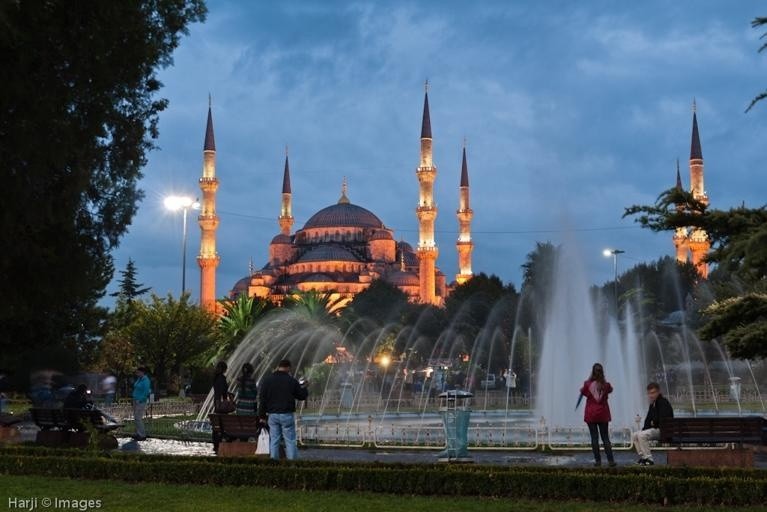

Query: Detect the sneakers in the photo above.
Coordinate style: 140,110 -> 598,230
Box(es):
636,458 -> 655,467
593,459 -> 618,468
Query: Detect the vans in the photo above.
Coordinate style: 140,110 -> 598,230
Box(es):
481,374 -> 497,389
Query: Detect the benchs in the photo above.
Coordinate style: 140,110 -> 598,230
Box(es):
207,412 -> 286,457
28,407 -> 126,447
656,417 -> 766,467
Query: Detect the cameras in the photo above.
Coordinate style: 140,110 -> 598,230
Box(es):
299,380 -> 306,385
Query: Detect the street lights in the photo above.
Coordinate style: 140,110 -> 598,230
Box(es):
163,195 -> 200,297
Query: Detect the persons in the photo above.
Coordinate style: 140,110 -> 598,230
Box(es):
502,368 -> 518,405
630,381 -> 674,468
131,367 -> 153,441
233,362 -> 258,414
258,360 -> 309,461
579,361 -> 619,467
211,360 -> 230,457
426,366 -> 466,406
100,370 -> 117,409
62,384 -> 88,426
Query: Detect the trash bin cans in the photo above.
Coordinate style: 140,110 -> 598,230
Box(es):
728,376 -> 742,402
340,383 -> 354,408
437,390 -> 474,464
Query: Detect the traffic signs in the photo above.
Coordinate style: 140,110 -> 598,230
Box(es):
602,248 -> 625,321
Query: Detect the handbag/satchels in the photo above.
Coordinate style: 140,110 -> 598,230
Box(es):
214,393 -> 237,414
